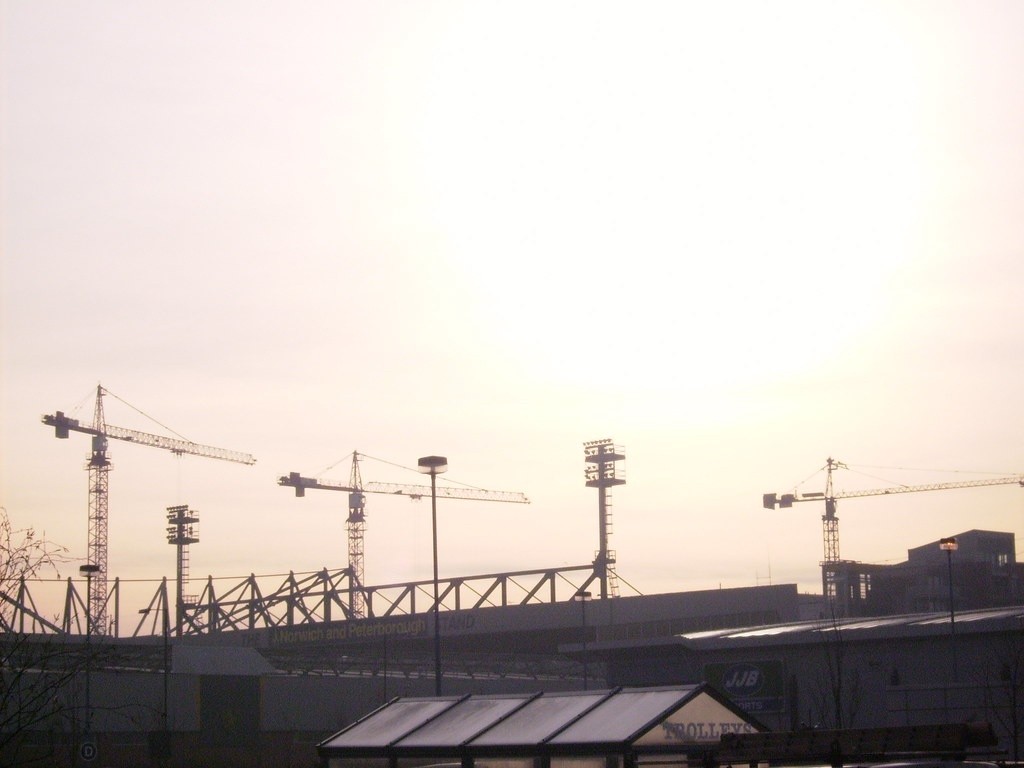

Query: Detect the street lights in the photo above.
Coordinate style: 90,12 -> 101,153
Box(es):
940,537 -> 960,680
75,563 -> 100,729
416,455 -> 449,694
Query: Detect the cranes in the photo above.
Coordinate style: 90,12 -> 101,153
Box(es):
44,382 -> 257,635
760,458 -> 1022,595
277,453 -> 533,622
